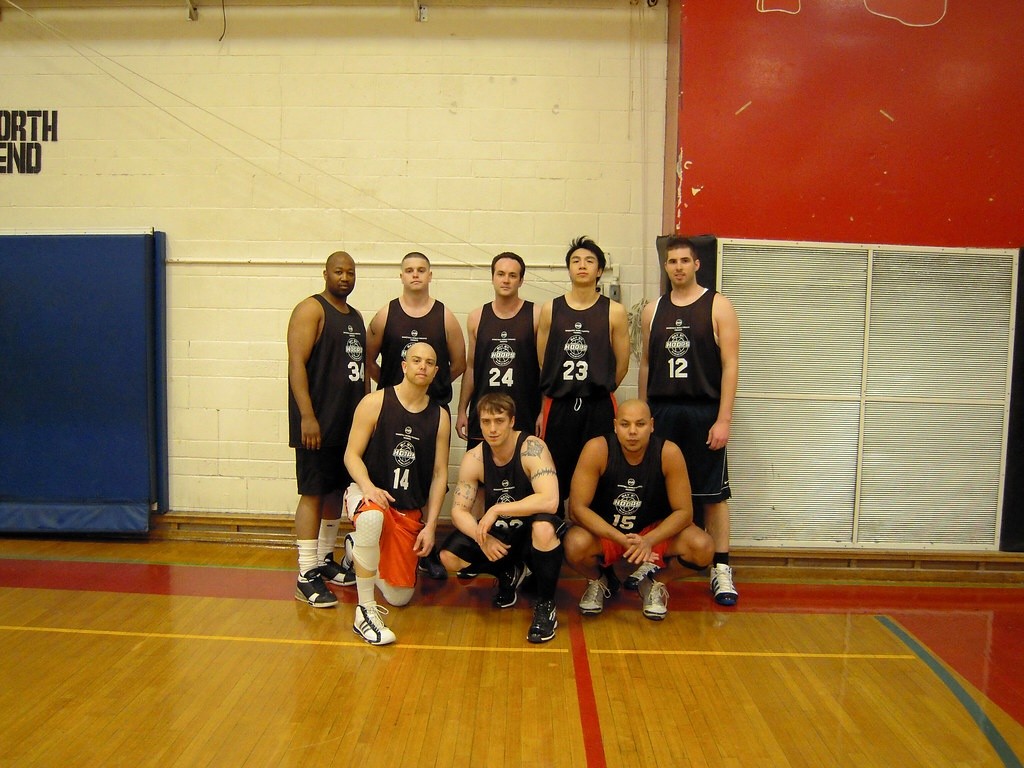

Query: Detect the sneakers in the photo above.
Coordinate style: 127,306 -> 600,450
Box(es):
527,596 -> 558,642
638,576 -> 670,621
319,551 -> 356,585
295,568 -> 338,607
493,561 -> 527,608
352,601 -> 396,645
419,547 -> 448,581
577,575 -> 610,616
623,561 -> 662,589
341,531 -> 358,572
601,567 -> 622,599
709,563 -> 738,605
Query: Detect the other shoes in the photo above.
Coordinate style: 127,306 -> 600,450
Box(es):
456,567 -> 478,578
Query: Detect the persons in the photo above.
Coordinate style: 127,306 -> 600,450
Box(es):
564,398 -> 715,620
341,341 -> 451,647
365,251 -> 467,577
455,253 -> 546,577
288,251 -> 372,610
439,392 -> 567,643
536,236 -> 739,606
